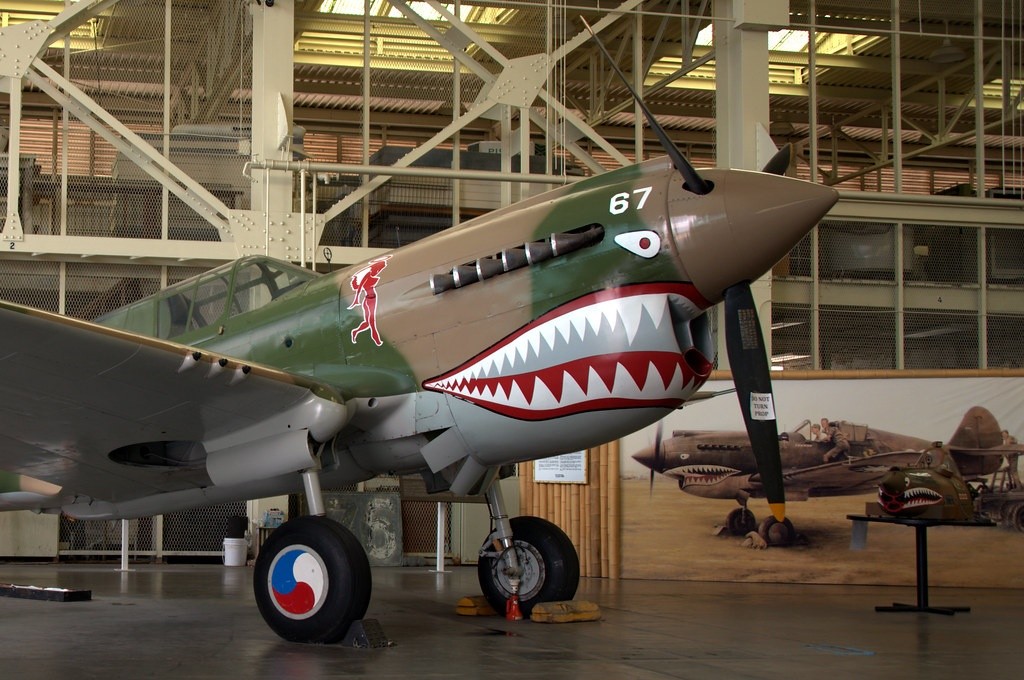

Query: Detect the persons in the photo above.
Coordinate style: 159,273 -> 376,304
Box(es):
812,418 -> 854,464
1002,430 -> 1023,492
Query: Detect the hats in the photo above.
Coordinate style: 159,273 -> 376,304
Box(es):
811,424 -> 820,429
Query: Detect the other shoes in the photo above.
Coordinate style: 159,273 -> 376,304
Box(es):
1011,487 -> 1024,492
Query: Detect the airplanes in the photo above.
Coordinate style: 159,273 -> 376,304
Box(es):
631,401 -> 1015,549
2,12 -> 843,647
